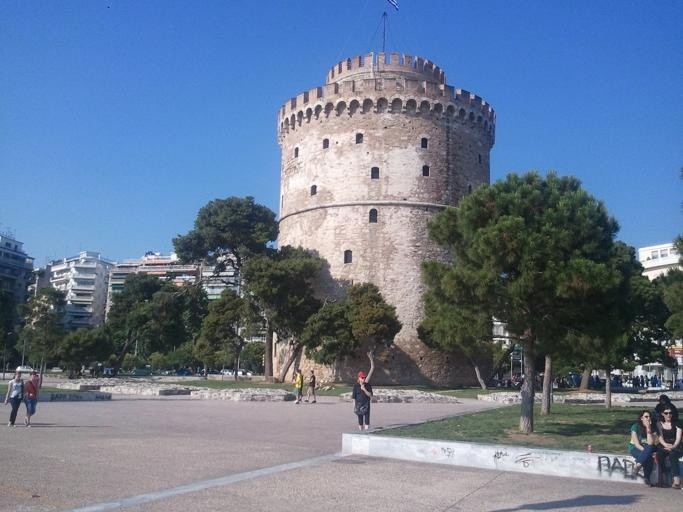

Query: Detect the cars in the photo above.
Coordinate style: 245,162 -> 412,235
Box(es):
16,366 -> 37,373
198,368 -> 253,376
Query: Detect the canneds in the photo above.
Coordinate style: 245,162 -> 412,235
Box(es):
587,444 -> 592,454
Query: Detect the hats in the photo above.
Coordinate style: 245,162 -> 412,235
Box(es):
359,372 -> 366,379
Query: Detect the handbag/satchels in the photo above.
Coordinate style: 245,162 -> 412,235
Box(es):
354,401 -> 369,415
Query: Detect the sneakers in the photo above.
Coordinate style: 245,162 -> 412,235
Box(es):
295,399 -> 316,404
645,480 -> 680,489
8,416 -> 31,427
633,466 -> 639,474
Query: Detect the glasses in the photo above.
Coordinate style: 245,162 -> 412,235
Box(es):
663,412 -> 672,415
645,416 -> 651,418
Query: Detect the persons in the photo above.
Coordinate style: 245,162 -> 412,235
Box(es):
653,394 -> 678,422
628,410 -> 654,488
632,374 -> 662,388
655,405 -> 683,488
351,370 -> 374,430
556,374 -> 601,388
22,371 -> 40,427
4,371 -> 24,427
291,370 -> 303,404
304,369 -> 317,404
